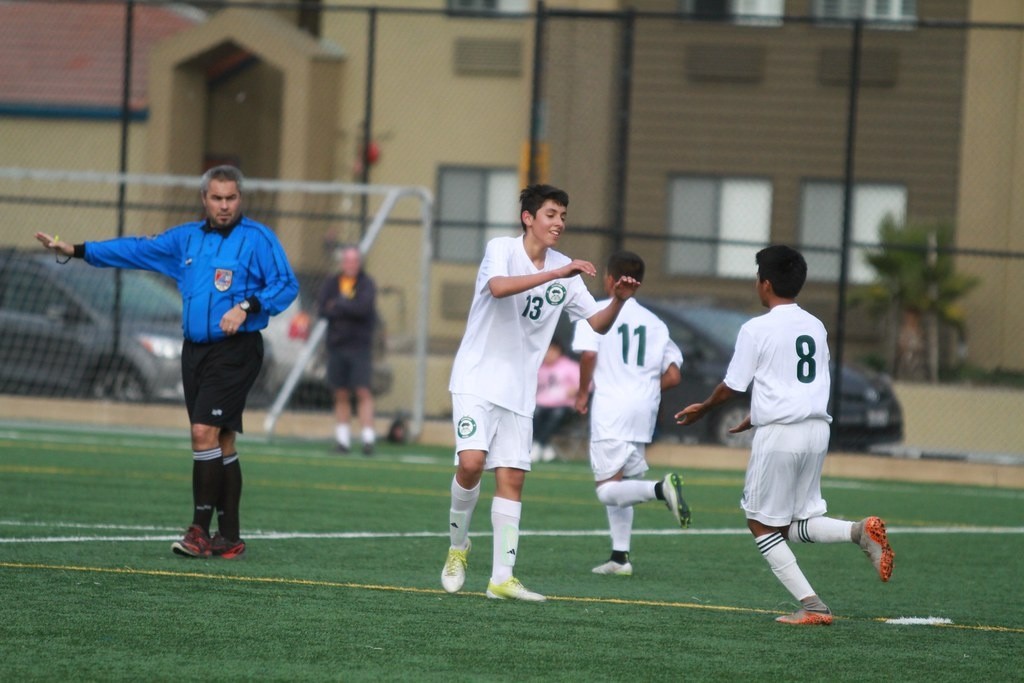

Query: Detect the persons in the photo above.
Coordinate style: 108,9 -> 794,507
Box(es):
531,338 -> 592,463
441,184 -> 641,602
34,165 -> 300,558
675,245 -> 894,624
573,250 -> 692,575
317,246 -> 377,456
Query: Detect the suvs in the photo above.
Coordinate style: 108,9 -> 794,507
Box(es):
259,270 -> 394,407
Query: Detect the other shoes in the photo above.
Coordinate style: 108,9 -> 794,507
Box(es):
530,441 -> 543,463
330,444 -> 351,454
362,443 -> 374,457
542,445 -> 556,463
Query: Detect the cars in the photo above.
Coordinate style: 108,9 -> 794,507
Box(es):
0,241 -> 278,409
551,295 -> 905,451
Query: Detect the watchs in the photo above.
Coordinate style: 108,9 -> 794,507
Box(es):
240,300 -> 250,311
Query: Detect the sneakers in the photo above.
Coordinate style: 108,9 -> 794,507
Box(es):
199,531 -> 246,559
857,516 -> 896,582
592,560 -> 632,576
662,473 -> 693,528
171,524 -> 212,558
440,537 -> 472,593
486,573 -> 546,603
775,607 -> 833,625
